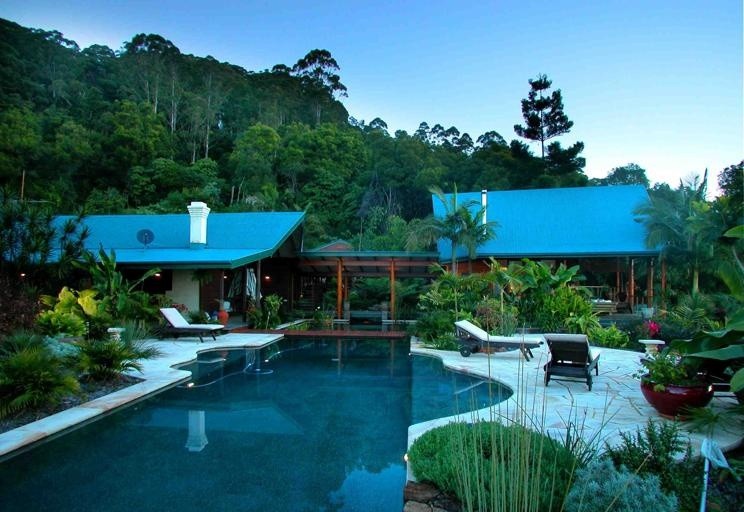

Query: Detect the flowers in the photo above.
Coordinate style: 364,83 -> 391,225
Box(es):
643,319 -> 661,339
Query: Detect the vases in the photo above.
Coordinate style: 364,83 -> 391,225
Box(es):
640,307 -> 654,318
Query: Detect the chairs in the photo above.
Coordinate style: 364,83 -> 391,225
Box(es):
452,317 -> 543,363
156,308 -> 224,344
541,333 -> 602,392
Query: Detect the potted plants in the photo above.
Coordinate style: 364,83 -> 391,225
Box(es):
637,353 -> 716,423
